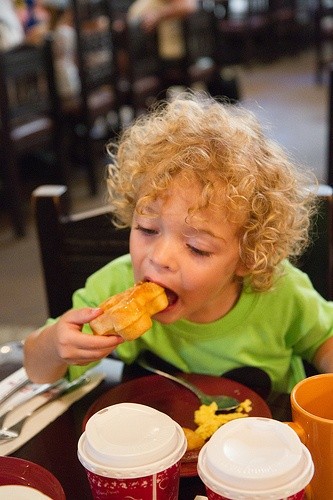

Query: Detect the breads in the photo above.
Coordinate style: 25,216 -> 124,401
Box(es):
89,282 -> 169,341
181,427 -> 206,450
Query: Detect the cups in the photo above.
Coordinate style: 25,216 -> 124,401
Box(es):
281,371 -> 333,500
196,416 -> 315,500
77,404 -> 188,500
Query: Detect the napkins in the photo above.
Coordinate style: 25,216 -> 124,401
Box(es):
0,366 -> 105,457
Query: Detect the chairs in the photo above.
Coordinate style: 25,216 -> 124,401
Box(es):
1,0 -> 333,243
31,185 -> 333,378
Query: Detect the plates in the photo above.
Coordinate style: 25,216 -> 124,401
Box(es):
82,369 -> 273,478
0,457 -> 65,500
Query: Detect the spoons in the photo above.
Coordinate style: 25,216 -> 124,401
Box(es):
135,357 -> 240,410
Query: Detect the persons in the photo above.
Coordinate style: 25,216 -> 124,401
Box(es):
0,0 -> 220,243
23,88 -> 333,421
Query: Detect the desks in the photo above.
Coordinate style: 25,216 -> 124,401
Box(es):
0,351 -> 292,500
